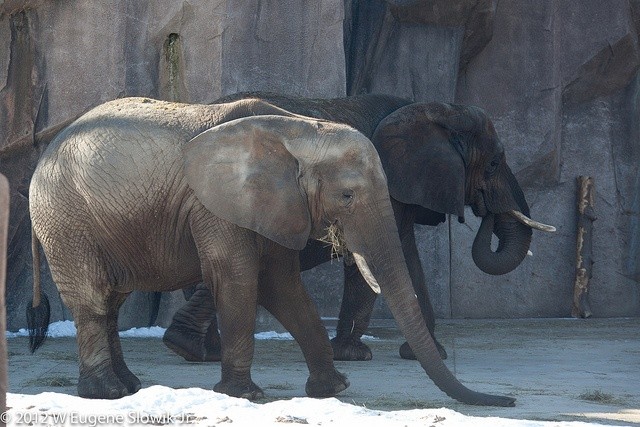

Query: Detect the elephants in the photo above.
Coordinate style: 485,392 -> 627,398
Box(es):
148,89 -> 561,362
25,93 -> 516,406
